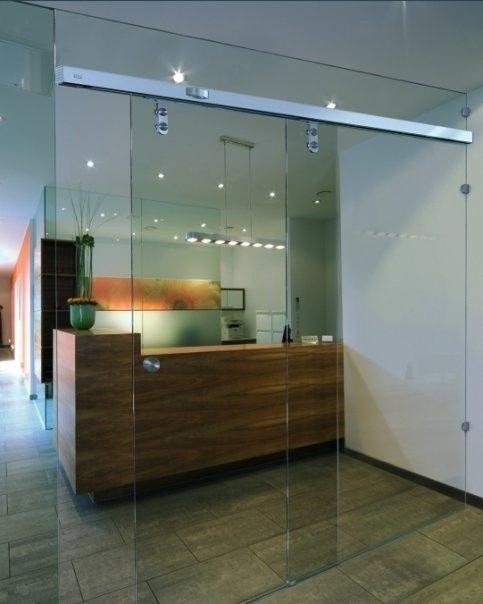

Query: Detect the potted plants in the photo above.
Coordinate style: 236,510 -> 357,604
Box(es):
67,234 -> 98,330
